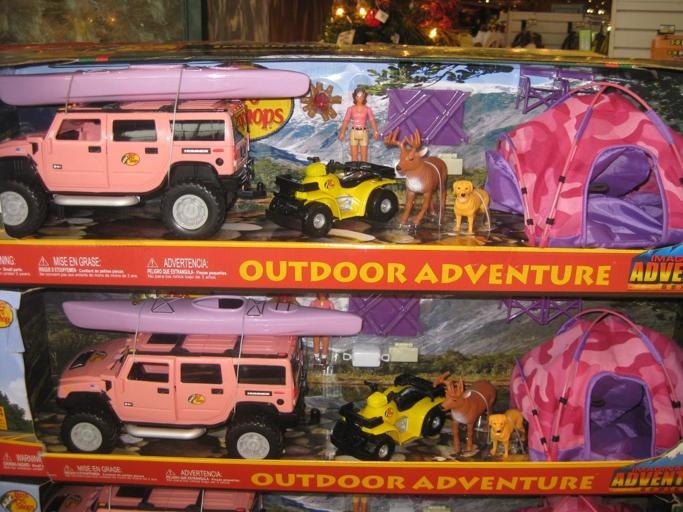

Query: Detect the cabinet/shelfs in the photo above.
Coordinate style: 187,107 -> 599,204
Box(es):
0,44 -> 683,511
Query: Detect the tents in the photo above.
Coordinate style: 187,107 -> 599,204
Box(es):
485,76 -> 683,247
509,306 -> 683,463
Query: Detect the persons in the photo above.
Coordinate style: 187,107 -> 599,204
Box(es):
309,293 -> 333,363
337,86 -> 378,162
